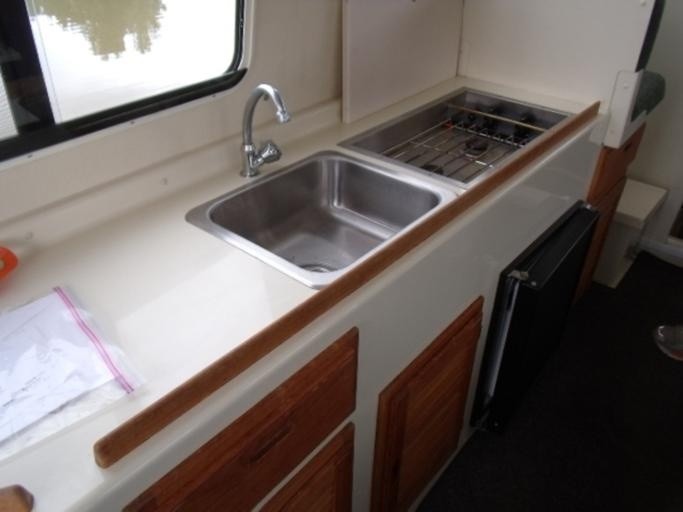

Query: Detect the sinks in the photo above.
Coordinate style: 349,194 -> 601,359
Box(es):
185,147 -> 460,288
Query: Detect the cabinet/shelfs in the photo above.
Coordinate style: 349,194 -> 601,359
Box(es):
118,325 -> 358,512
370,295 -> 484,512
570,121 -> 645,304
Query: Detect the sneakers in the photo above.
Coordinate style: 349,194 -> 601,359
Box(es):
651,324 -> 683,361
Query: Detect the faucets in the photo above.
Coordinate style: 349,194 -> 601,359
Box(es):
241,82 -> 290,145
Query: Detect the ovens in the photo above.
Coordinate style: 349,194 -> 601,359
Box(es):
474,203 -> 604,434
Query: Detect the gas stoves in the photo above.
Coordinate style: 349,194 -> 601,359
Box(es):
346,86 -> 566,186
